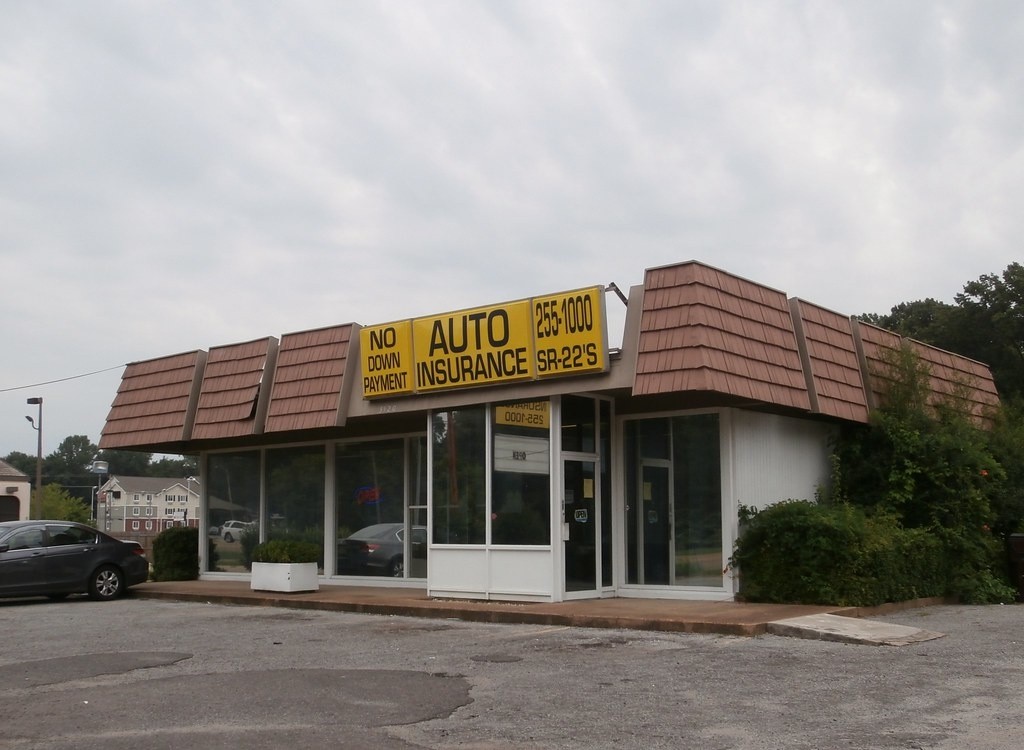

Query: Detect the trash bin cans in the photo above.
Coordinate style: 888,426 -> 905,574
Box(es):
1000,532 -> 1024,594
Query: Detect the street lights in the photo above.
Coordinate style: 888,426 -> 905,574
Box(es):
25,397 -> 43,519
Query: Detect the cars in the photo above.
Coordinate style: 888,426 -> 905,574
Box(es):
0,520 -> 149,601
340,522 -> 428,577
208,521 -> 252,543
